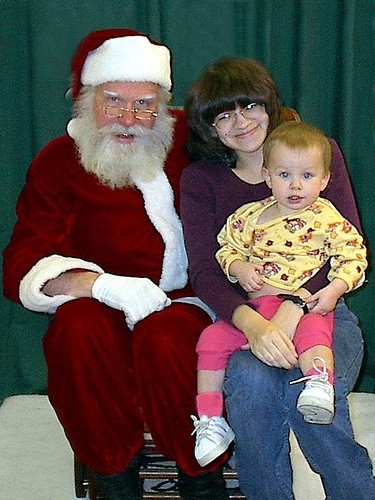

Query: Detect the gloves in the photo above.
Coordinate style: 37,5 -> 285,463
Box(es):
92,272 -> 171,330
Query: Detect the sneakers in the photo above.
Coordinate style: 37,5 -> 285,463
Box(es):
190,414 -> 235,467
290,357 -> 334,424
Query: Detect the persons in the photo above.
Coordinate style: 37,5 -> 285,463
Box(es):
190,121 -> 369,467
179,55 -> 375,499
1,29 -> 232,500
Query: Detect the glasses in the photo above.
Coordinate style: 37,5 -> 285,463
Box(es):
210,102 -> 265,131
95,103 -> 158,122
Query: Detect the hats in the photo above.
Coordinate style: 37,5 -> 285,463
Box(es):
66,27 -> 172,138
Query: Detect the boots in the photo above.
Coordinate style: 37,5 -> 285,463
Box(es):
176,465 -> 230,500
84,462 -> 145,500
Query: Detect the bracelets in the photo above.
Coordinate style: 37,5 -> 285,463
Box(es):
277,294 -> 308,314
